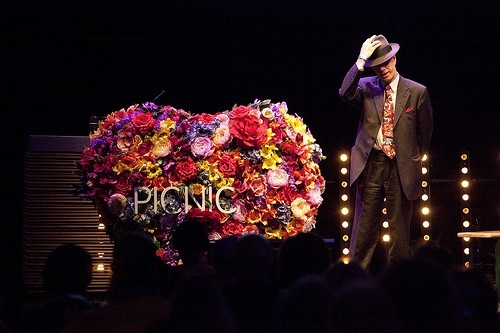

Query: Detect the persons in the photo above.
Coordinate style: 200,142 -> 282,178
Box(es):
0,220 -> 500,333
338,35 -> 432,271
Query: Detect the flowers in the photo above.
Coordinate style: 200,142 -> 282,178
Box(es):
74,100 -> 327,266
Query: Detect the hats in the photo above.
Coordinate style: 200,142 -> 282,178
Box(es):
365,34 -> 400,68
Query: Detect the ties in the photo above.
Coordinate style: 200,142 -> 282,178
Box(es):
382,84 -> 397,160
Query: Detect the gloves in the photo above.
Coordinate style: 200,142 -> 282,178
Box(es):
357,35 -> 382,61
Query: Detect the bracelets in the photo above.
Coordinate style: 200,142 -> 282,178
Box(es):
357,57 -> 367,62
